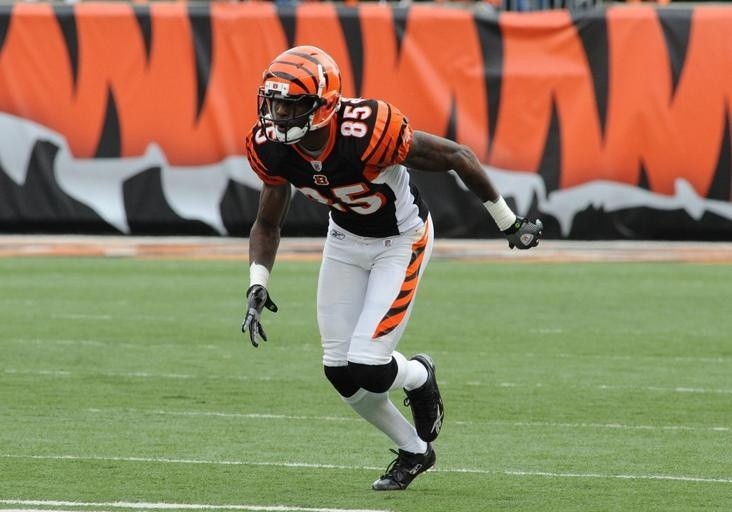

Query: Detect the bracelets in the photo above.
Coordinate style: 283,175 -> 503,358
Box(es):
484,196 -> 516,232
248,263 -> 270,289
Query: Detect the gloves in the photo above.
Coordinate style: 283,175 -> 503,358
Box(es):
500,216 -> 544,250
242,285 -> 277,348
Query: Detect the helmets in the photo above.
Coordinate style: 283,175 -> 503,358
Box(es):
255,43 -> 343,145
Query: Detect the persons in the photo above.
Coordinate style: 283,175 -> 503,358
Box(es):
241,45 -> 544,489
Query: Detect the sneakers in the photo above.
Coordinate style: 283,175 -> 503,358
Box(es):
402,353 -> 445,443
371,443 -> 437,490
